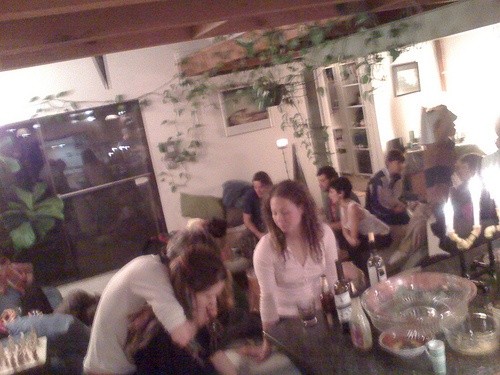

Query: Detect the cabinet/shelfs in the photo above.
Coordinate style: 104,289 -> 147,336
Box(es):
313,58 -> 389,193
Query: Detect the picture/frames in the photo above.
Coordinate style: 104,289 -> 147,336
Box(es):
219,83 -> 271,135
392,62 -> 421,98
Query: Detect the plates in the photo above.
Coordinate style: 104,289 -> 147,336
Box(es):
378,331 -> 434,356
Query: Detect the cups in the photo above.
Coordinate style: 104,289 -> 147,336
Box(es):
0,331 -> 39,371
295,297 -> 317,326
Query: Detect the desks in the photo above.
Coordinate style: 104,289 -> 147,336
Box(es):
263,238 -> 500,375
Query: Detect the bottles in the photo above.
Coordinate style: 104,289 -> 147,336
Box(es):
349,293 -> 372,355
366,232 -> 387,285
333,259 -> 353,331
319,274 -> 338,328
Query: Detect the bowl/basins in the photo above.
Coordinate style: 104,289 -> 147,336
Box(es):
438,306 -> 500,354
360,272 -> 477,338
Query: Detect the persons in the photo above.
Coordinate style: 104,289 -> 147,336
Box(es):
51,147 -> 140,231
0,151 -> 500,375
